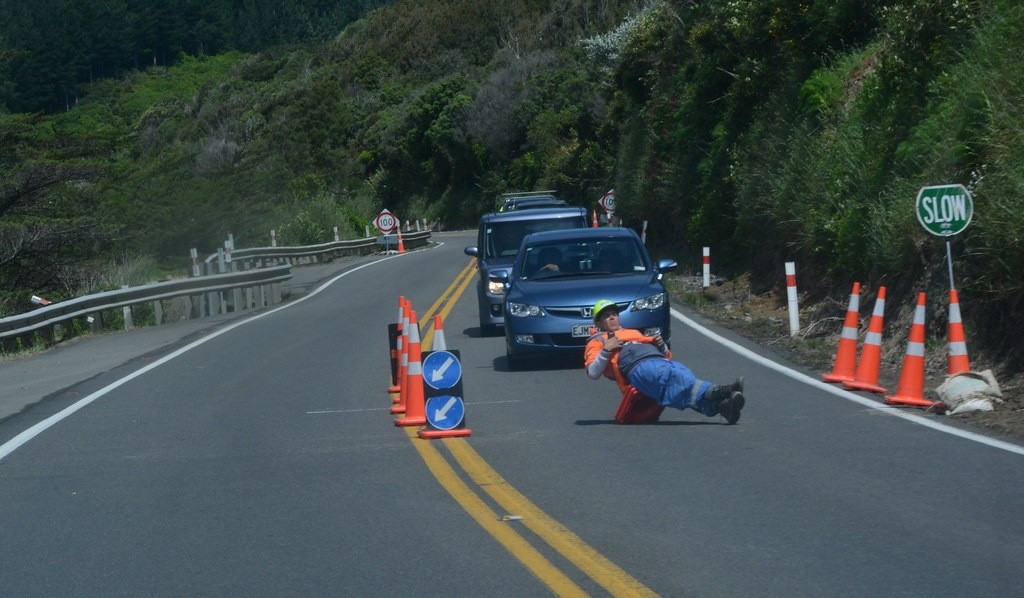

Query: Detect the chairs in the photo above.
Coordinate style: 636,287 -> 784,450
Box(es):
537,248 -> 563,270
597,248 -> 628,270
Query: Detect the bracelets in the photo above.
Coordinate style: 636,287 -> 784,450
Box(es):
603,348 -> 613,352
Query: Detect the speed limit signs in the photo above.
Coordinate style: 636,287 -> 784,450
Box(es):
372,208 -> 400,236
598,188 -> 617,217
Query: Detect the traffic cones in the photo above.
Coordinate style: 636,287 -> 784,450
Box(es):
592,210 -> 599,228
946,289 -> 970,375
397,229 -> 406,253
843,287 -> 888,394
416,314 -> 472,439
822,280 -> 861,384
885,291 -> 940,406
388,295 -> 425,428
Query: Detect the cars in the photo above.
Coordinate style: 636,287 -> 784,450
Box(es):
465,189 -> 588,335
488,225 -> 677,362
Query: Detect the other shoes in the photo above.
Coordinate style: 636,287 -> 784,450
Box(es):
711,376 -> 743,400
715,391 -> 745,424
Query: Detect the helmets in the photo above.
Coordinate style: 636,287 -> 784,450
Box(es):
593,300 -> 618,324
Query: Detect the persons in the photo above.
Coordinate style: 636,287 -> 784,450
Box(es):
584,299 -> 746,424
599,257 -> 614,271
536,247 -> 569,273
